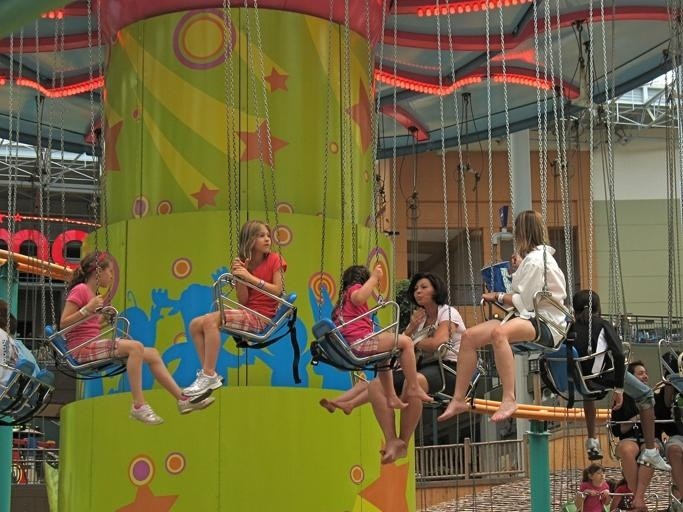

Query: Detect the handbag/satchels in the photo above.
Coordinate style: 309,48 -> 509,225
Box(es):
408,326 -> 447,364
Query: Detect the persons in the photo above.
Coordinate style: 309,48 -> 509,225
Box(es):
610,360 -> 659,512
332,265 -> 433,408
652,353 -> 679,460
367,273 -> 467,468
59,252 -> 215,424
564,290 -> 673,471
608,478 -> 635,512
579,464 -> 610,512
0,299 -> 54,424
654,351 -> 683,511
437,210 -> 569,421
319,380 -> 372,415
181,219 -> 288,397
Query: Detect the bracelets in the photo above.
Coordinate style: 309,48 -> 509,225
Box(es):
498,293 -> 507,304
255,278 -> 264,289
79,306 -> 92,318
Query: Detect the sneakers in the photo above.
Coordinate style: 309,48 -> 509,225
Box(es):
129,404 -> 163,425
586,438 -> 603,460
637,448 -> 672,471
178,369 -> 223,414
